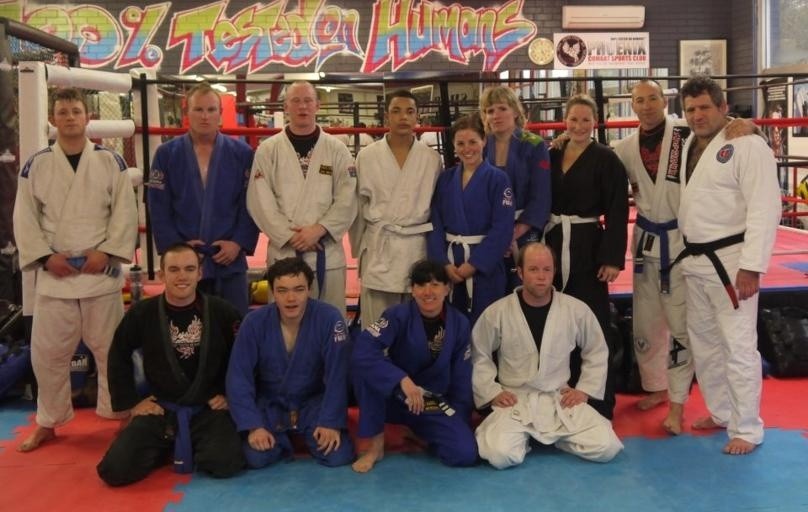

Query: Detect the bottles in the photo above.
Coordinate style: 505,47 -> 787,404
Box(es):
126,265 -> 143,304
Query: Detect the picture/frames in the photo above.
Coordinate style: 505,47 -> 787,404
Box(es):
679,38 -> 727,119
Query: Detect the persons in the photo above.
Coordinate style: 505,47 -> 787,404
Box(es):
427,115 -> 516,322
348,89 -> 444,330
346,259 -> 478,473
328,120 -> 374,155
678,79 -> 783,453
246,79 -> 356,321
469,241 -> 623,470
95,241 -> 247,486
13,88 -> 137,451
226,257 -> 357,468
546,94 -> 628,421
551,79 -> 769,434
480,85 -> 550,296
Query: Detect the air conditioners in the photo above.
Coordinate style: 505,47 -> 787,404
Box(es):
561,4 -> 645,30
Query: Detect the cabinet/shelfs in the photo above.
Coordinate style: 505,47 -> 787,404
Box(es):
1,17 -> 81,344
